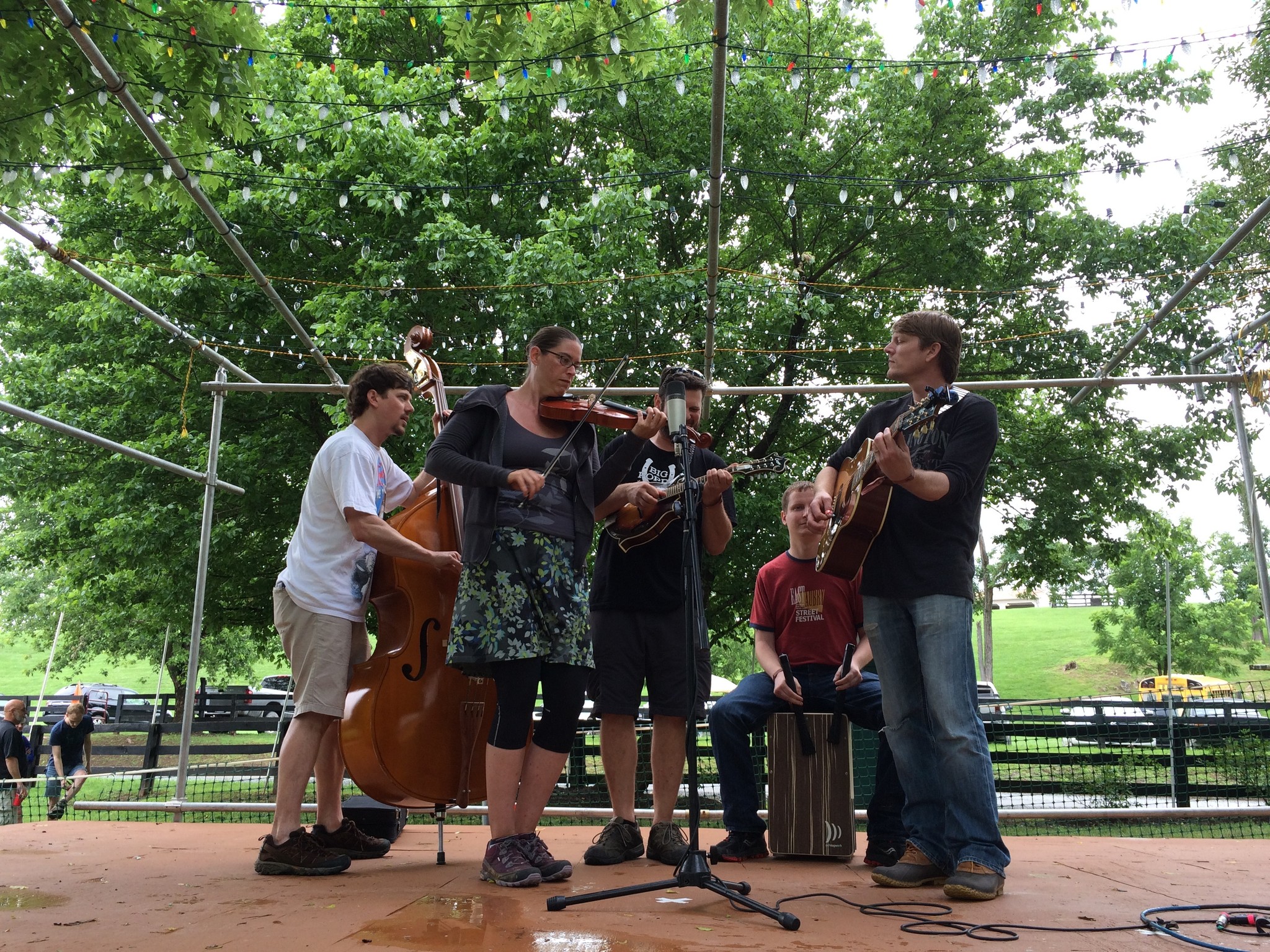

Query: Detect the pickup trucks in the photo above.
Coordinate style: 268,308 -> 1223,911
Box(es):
193,674 -> 296,735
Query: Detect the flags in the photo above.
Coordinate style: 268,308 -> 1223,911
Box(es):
72,680 -> 82,703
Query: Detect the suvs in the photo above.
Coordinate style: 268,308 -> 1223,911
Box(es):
42,682 -> 173,725
976,681 -> 1013,746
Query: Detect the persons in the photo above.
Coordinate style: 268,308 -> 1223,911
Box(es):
804,310 -> 1011,901
255,362 -> 462,874
583,365 -> 736,865
0,699 -> 34,826
424,327 -> 668,886
709,480 -> 910,866
43,703 -> 94,821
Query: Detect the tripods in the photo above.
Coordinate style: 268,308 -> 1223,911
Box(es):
545,433 -> 802,931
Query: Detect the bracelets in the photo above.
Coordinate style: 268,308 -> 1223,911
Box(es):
773,668 -> 783,682
893,468 -> 915,484
700,494 -> 723,509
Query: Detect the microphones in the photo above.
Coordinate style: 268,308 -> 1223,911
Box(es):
666,380 -> 687,458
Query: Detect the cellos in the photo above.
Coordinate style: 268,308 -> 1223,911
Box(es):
340,326 -> 538,864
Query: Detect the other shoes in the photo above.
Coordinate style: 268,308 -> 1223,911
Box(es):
46,803 -> 65,820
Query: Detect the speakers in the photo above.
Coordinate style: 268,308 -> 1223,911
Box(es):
340,795 -> 409,842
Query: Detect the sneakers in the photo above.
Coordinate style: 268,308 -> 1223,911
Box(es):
710,831 -> 769,860
480,834 -> 543,888
862,842 -> 908,868
583,817 -> 645,865
305,817 -> 390,859
253,827 -> 352,876
943,860 -> 1005,900
646,820 -> 690,866
871,843 -> 949,887
519,830 -> 573,881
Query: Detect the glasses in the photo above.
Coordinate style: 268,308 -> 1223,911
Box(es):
541,349 -> 583,372
14,707 -> 26,712
664,367 -> 706,380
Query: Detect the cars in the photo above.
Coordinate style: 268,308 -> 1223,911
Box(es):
1060,696 -> 1157,750
1181,698 -> 1267,719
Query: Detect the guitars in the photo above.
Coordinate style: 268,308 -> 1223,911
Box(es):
810,396 -> 942,585
597,450 -> 792,557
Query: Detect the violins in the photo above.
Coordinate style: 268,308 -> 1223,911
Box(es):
538,392 -> 713,451
